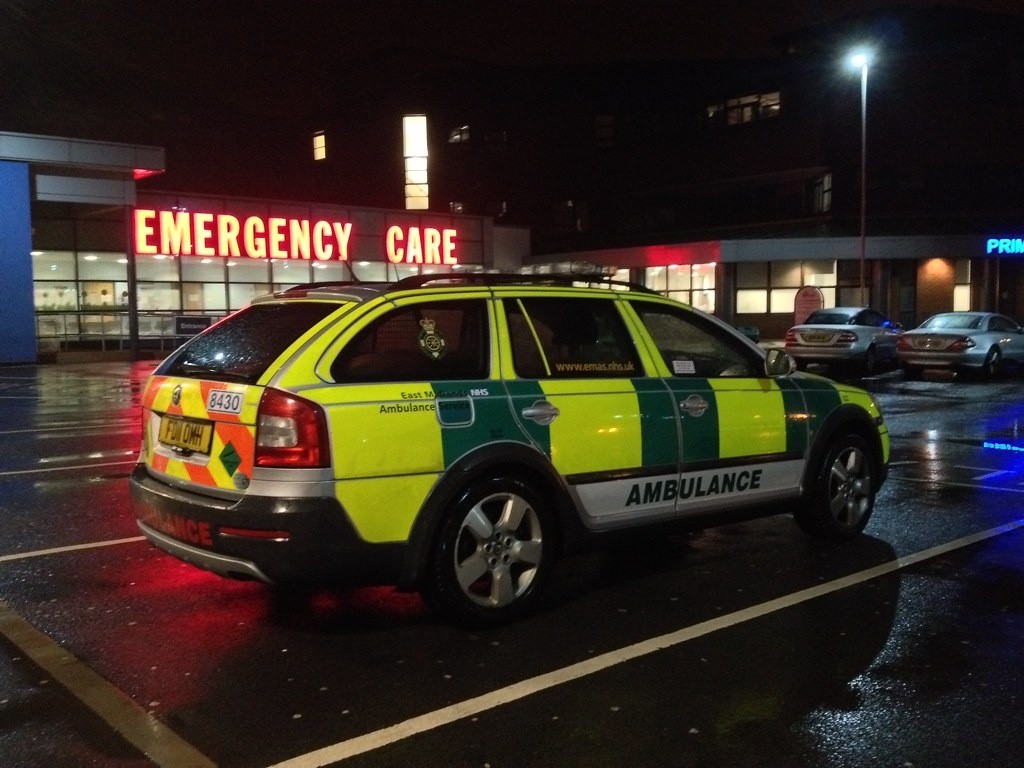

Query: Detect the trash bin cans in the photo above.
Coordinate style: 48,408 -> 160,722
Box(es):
738,326 -> 760,344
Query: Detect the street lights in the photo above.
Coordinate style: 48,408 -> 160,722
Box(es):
850,52 -> 870,309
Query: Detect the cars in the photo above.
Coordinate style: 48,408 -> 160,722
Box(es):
783,306 -> 908,371
895,310 -> 1024,377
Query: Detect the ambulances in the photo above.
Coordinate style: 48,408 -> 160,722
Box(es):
128,273 -> 894,623
